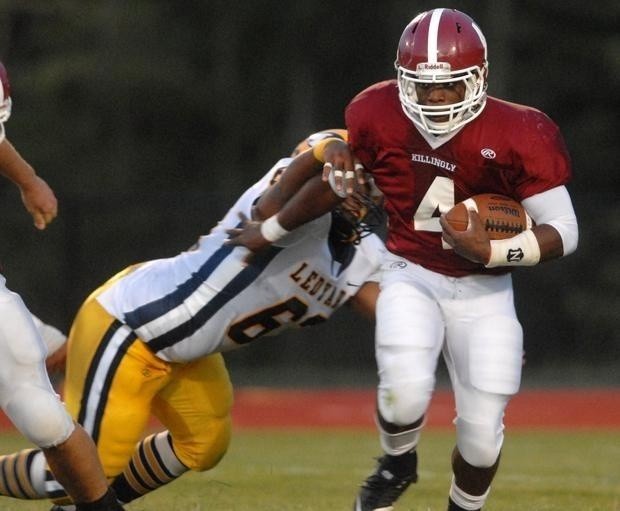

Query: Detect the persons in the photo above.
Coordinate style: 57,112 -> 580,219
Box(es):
0,61 -> 127,511
223,6 -> 584,511
0,128 -> 388,507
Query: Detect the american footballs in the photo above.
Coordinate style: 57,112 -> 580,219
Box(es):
445,193 -> 535,240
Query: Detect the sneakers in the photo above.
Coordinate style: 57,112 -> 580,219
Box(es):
352,446 -> 418,510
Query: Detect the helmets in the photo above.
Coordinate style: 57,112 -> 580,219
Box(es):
394,7 -> 489,135
275,127 -> 389,245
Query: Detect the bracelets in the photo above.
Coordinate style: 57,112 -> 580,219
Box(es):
260,212 -> 291,246
482,227 -> 540,268
